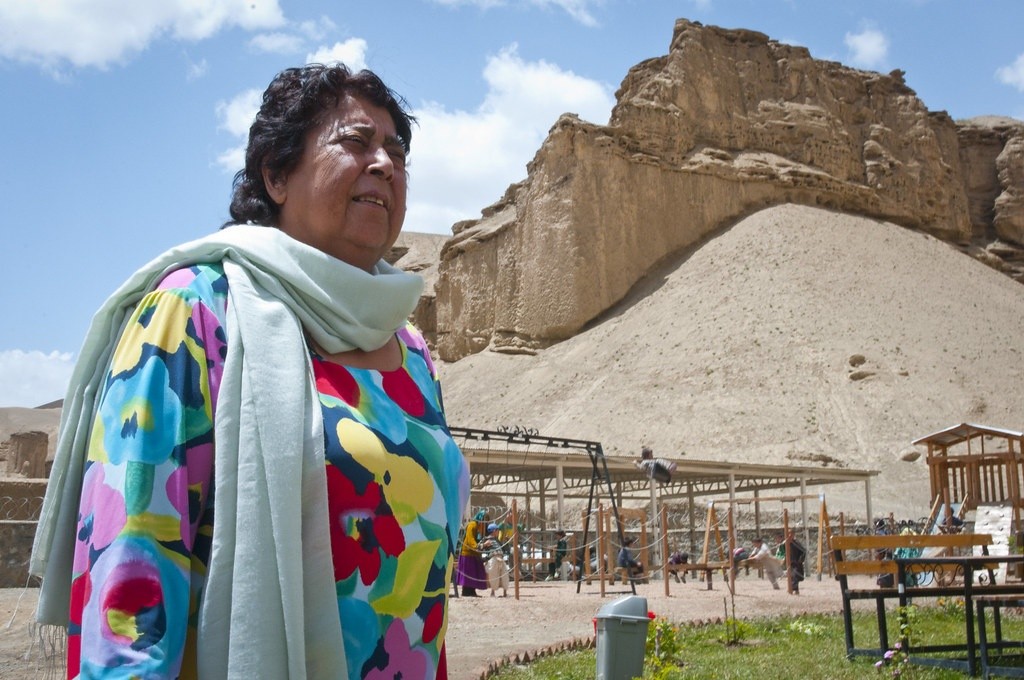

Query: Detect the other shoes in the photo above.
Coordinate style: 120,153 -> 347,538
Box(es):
681,577 -> 686,584
554,571 -> 560,579
545,575 -> 554,581
673,575 -> 680,584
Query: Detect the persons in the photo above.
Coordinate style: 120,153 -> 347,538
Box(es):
668,552 -> 689,584
634,449 -> 680,484
726,547 -> 749,575
875,519 -> 918,588
545,531 -> 567,581
741,535 -> 783,589
943,508 -> 962,527
457,509 -> 494,596
476,523 -> 509,596
777,529 -> 808,594
27,60 -> 472,679
616,536 -> 644,584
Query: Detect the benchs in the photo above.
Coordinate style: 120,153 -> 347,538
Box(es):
829,533 -> 1024,680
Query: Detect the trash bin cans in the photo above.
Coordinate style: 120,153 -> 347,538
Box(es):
591,595 -> 656,680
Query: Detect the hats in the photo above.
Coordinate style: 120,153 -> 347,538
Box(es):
487,523 -> 501,531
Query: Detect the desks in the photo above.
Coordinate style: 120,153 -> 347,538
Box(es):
893,553 -> 1024,678
